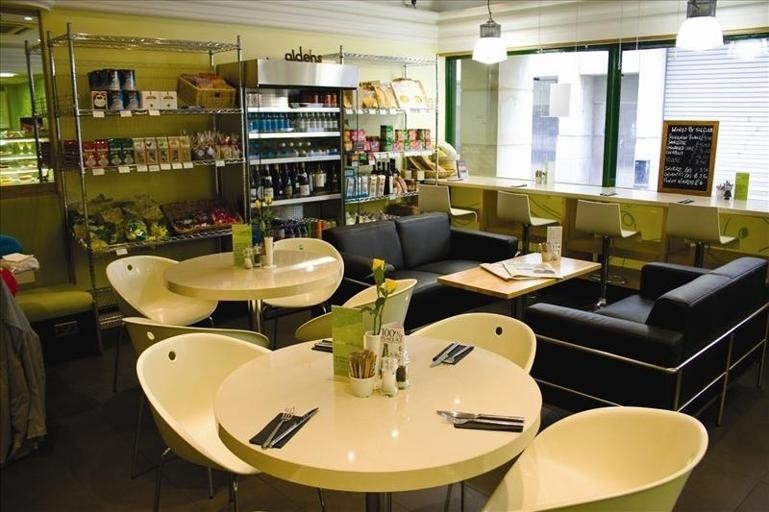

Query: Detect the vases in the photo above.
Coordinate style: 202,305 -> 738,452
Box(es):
262,235 -> 274,266
361,330 -> 381,392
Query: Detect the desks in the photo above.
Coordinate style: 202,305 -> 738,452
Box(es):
213,336 -> 545,512
438,252 -> 603,324
160,248 -> 342,331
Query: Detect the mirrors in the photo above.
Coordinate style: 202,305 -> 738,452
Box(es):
0,6 -> 58,203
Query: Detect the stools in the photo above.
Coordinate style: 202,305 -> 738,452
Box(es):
575,198 -> 639,312
132,329 -> 328,511
666,200 -> 741,269
494,189 -> 560,255
416,183 -> 479,232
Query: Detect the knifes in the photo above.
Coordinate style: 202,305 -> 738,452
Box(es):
269,406 -> 320,447
429,343 -> 460,369
436,409 -> 524,423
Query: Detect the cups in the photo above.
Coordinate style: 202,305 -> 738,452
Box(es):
348,371 -> 376,397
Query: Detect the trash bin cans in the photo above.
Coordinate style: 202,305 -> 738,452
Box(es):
634,160 -> 650,184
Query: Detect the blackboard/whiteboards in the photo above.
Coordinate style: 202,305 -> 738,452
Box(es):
657,121 -> 719,197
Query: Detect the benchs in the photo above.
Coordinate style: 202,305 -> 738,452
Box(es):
12,282 -> 95,324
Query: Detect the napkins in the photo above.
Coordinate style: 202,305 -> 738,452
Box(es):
250,412 -> 307,451
431,341 -> 475,367
312,338 -> 332,355
453,411 -> 527,435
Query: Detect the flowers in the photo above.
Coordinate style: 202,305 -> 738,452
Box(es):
252,197 -> 276,233
353,254 -> 398,333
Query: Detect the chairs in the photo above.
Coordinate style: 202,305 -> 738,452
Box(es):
405,306 -> 539,512
479,402 -> 712,512
249,236 -> 346,337
290,277 -> 420,339
120,315 -> 271,480
104,253 -> 217,393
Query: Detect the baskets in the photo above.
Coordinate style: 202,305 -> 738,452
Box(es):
410,168 -> 455,178
177,76 -> 237,108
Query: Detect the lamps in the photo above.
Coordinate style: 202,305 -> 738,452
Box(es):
473,1 -> 509,65
675,1 -> 725,52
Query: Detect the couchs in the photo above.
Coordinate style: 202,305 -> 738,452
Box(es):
318,211 -> 519,333
520,255 -> 768,428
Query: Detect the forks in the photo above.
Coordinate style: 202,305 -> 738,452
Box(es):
439,344 -> 473,364
441,411 -> 528,425
261,404 -> 296,449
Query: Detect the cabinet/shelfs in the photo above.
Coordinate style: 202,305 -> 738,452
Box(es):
47,21 -> 248,356
307,45 -> 439,227
0,130 -> 50,179
216,44 -> 358,320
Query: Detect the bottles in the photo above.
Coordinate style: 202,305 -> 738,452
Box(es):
243,244 -> 262,269
371,161 -> 393,194
382,346 -> 410,396
248,112 -> 339,239
